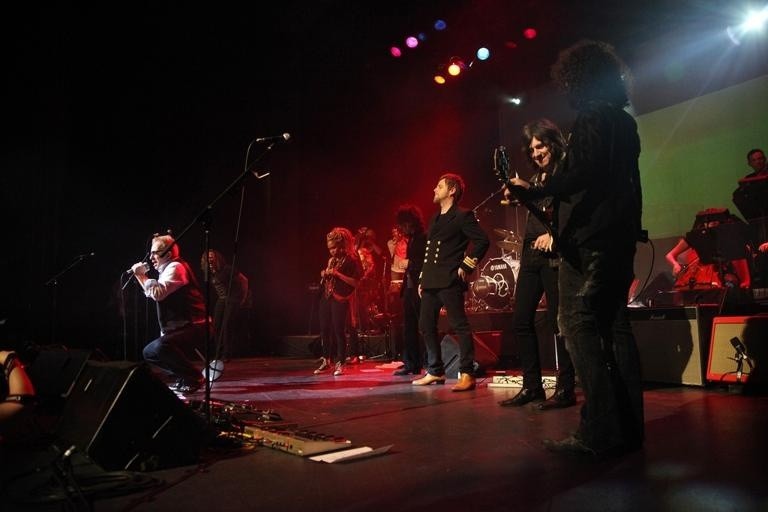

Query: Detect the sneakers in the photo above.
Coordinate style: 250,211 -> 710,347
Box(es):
167,376 -> 206,393
313,360 -> 334,375
333,361 -> 346,375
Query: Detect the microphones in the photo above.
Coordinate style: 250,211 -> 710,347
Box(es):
80,252 -> 95,258
126,262 -> 150,275
256,133 -> 291,144
730,336 -> 750,362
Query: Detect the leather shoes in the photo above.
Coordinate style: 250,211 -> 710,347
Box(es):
411,372 -> 446,386
498,388 -> 546,408
451,373 -> 476,391
538,392 -> 577,410
394,361 -> 424,376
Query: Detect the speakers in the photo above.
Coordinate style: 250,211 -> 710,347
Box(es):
626,304 -> 719,387
706,313 -> 768,385
51,368 -> 206,468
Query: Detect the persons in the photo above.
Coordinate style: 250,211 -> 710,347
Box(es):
132,235 -> 216,397
313,227 -> 365,376
346,225 -> 411,367
498,118 -> 576,411
542,39 -> 645,457
665,209 -> 740,290
0,350 -> 35,419
201,249 -> 249,363
396,210 -> 428,376
411,173 -> 490,392
733,149 -> 768,221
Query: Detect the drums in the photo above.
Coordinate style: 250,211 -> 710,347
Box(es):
481,256 -> 521,310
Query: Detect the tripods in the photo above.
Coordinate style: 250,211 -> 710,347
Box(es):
195,231 -> 240,448
345,289 -> 377,357
368,281 -> 400,360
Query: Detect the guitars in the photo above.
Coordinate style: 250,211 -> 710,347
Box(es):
494,146 -> 552,235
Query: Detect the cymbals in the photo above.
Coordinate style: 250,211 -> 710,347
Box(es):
494,228 -> 522,242
496,240 -> 522,253
